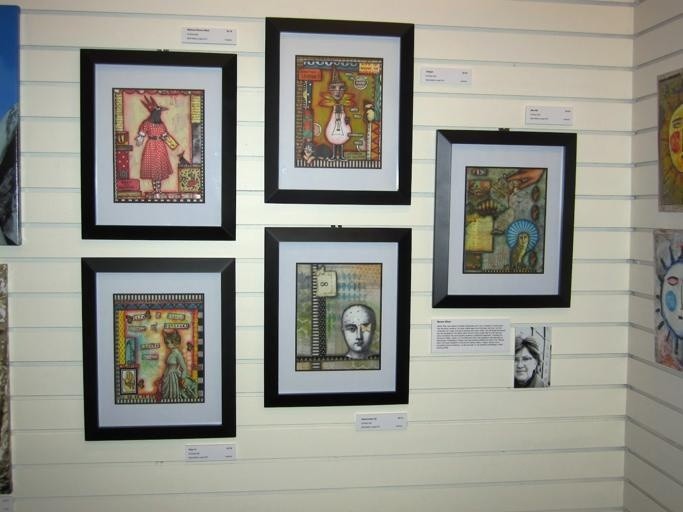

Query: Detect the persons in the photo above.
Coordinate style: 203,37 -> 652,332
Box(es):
514,336 -> 547,388
340,303 -> 378,360
156,328 -> 198,399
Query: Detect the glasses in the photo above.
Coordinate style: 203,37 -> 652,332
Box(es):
514,357 -> 533,362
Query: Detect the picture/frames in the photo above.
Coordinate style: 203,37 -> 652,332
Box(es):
264,16 -> 415,206
262,225 -> 413,409
0,265 -> 14,492
431,130 -> 579,308
80,48 -> 237,241
80,255 -> 237,443
0,4 -> 21,244
